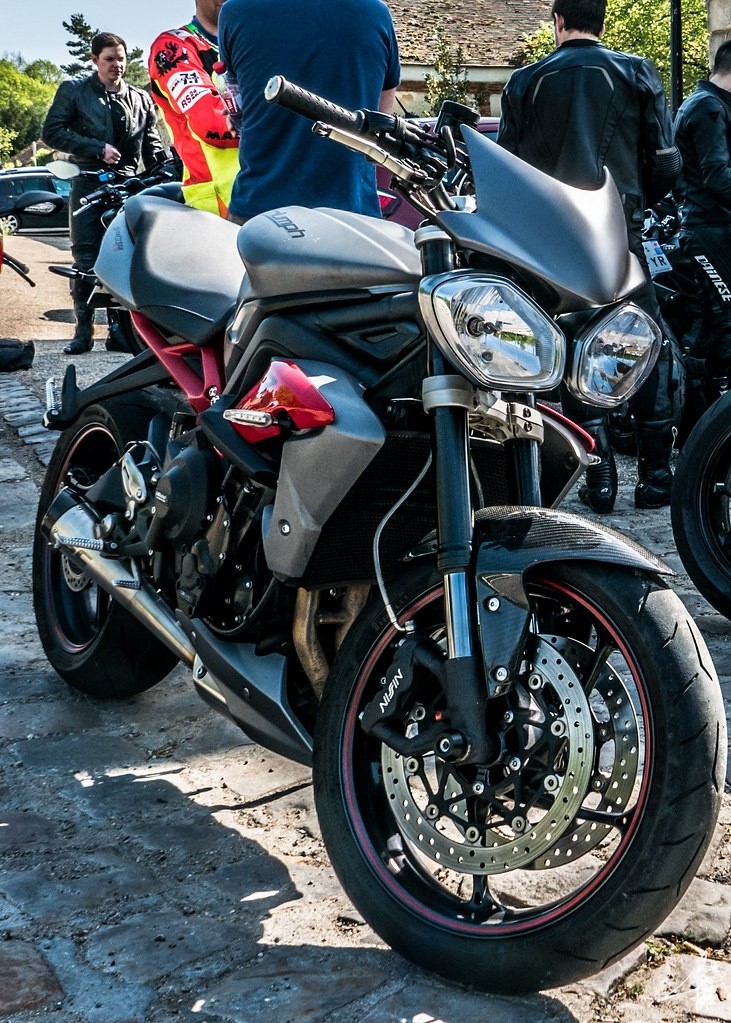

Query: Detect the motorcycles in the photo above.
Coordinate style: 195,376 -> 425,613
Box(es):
44,153 -> 183,356
31,76 -> 728,994
608,194 -> 731,623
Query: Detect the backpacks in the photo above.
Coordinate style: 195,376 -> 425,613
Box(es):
0,338 -> 35,372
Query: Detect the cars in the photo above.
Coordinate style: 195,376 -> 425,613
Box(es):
375,117 -> 500,231
0,167 -> 70,236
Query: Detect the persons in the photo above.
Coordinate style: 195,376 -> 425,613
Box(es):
670,38 -> 731,435
217,0 -> 401,226
146,0 -> 241,219
42,31 -> 167,355
495,0 -> 685,512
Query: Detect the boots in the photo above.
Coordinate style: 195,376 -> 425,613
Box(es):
633,419 -> 679,509
104,303 -> 144,353
63,303 -> 97,354
575,419 -> 619,513
